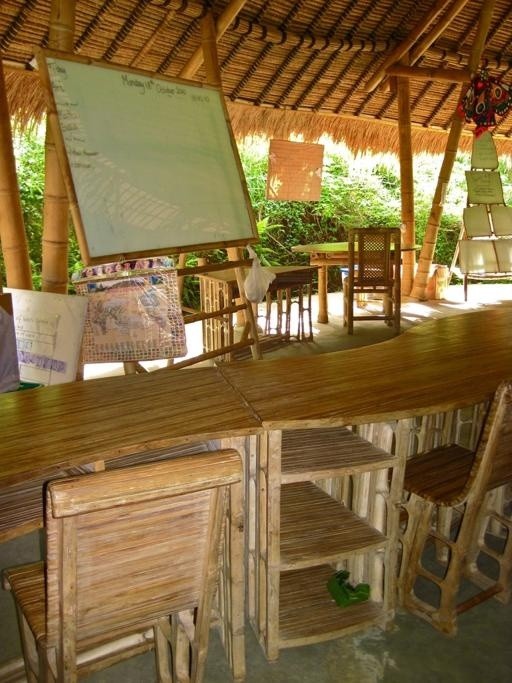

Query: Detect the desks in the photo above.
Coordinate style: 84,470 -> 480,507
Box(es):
206,241 -> 422,341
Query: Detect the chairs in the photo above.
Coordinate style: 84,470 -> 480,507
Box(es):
345,227 -> 404,338
